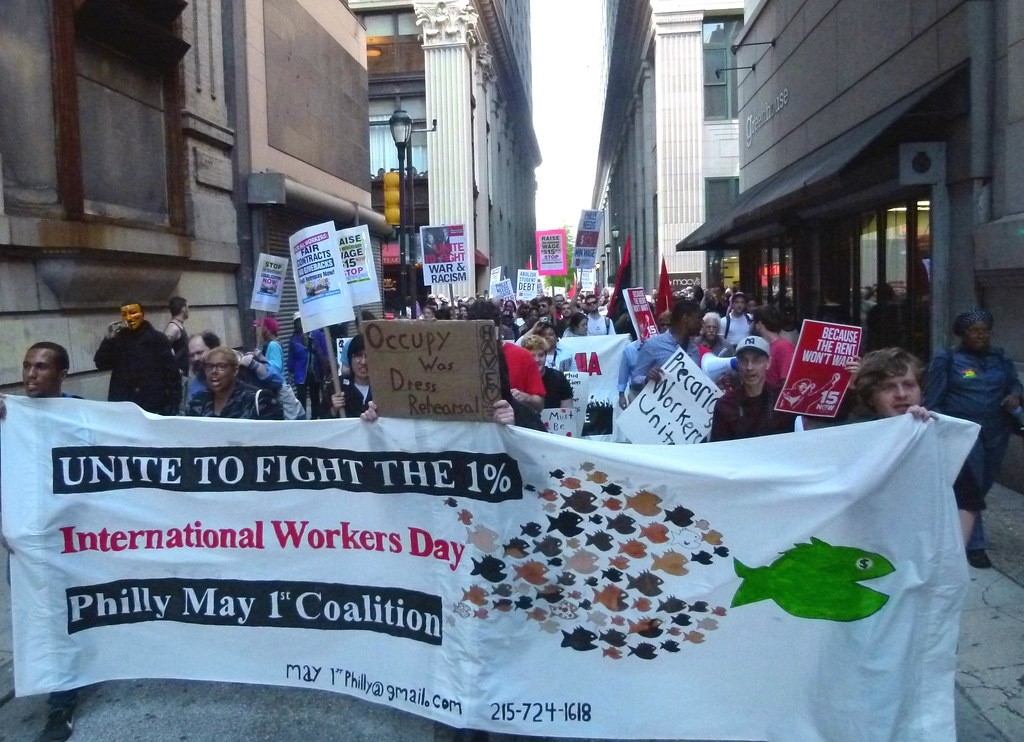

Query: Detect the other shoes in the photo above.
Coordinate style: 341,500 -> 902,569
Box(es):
967,549 -> 992,567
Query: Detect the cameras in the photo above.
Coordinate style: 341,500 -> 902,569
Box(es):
540,317 -> 548,322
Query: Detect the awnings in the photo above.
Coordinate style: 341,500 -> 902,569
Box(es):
675,63 -> 968,252
475,249 -> 489,265
382,242 -> 400,265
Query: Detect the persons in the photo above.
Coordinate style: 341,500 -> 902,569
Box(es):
925,314 -> 1024,570
859,282 -> 909,342
0,342 -> 100,742
94,281 -> 926,742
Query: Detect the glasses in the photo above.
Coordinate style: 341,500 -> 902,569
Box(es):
587,301 -> 596,306
205,363 -> 232,371
538,304 -> 546,308
351,353 -> 367,362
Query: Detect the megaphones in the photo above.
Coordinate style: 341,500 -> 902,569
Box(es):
701,352 -> 739,383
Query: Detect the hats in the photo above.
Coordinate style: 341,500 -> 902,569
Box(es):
293,312 -> 301,322
735,335 -> 771,358
954,310 -> 993,336
501,311 -> 513,319
732,292 -> 747,301
253,318 -> 278,334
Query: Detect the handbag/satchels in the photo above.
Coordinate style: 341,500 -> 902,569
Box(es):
319,354 -> 330,377
1008,404 -> 1024,437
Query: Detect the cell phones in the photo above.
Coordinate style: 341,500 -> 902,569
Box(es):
113,321 -> 128,331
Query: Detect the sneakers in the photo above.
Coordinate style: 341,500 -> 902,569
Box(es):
45,707 -> 76,742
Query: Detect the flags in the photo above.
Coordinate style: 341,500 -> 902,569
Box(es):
594,282 -> 598,295
654,257 -> 674,322
567,276 -> 576,297
607,234 -> 631,317
525,259 -> 531,270
